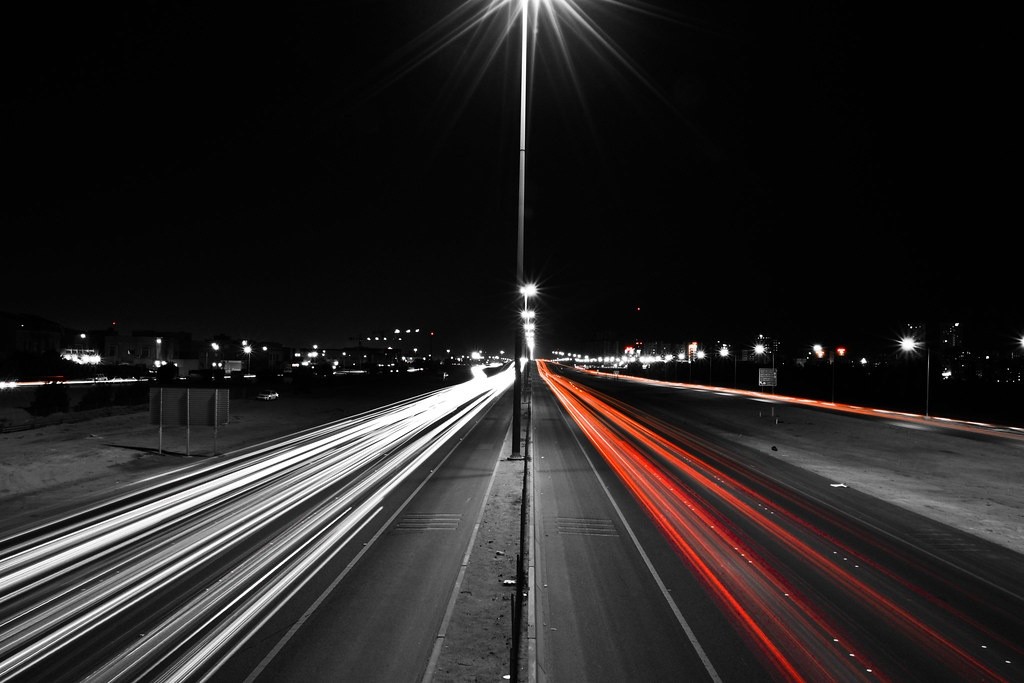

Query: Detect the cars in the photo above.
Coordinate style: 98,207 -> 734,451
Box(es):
258,389 -> 280,401
88,374 -> 108,381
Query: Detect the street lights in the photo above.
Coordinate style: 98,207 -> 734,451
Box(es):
663,345 -> 775,396
900,336 -> 930,416
